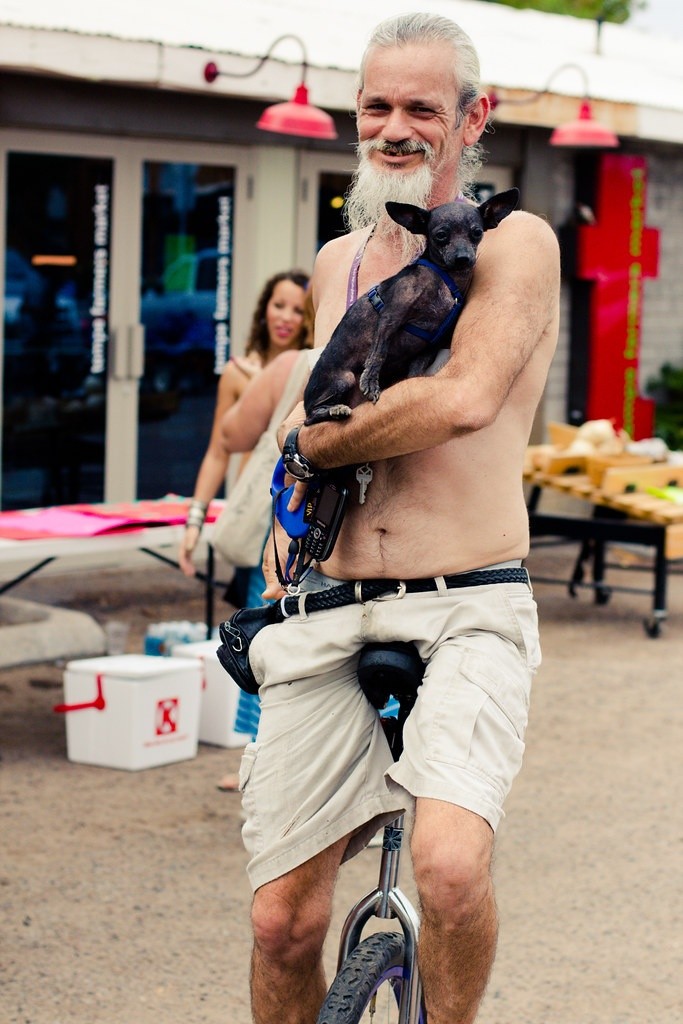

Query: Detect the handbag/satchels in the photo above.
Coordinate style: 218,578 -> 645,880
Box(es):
209,349 -> 313,568
217,606 -> 269,694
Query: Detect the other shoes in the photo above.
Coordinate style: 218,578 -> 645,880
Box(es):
219,776 -> 242,792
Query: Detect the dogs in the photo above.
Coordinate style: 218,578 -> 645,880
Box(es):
301,187 -> 521,418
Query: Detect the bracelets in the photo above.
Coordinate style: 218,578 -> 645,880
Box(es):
190,501 -> 208,509
188,509 -> 205,518
185,518 -> 203,528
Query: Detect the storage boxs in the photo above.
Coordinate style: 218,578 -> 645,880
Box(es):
54,640 -> 252,771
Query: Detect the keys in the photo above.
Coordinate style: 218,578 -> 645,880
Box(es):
356,465 -> 374,505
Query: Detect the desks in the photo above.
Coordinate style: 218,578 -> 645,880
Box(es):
516,443 -> 683,638
0,498 -> 228,641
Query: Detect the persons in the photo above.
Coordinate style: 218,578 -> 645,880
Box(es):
239,14 -> 560,1024
178,272 -> 315,791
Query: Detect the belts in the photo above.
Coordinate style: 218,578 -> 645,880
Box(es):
270,567 -> 529,620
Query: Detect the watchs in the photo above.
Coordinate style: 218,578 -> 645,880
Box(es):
281,424 -> 317,482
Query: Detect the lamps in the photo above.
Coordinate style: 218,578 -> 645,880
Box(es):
489,64 -> 619,147
205,33 -> 338,140
